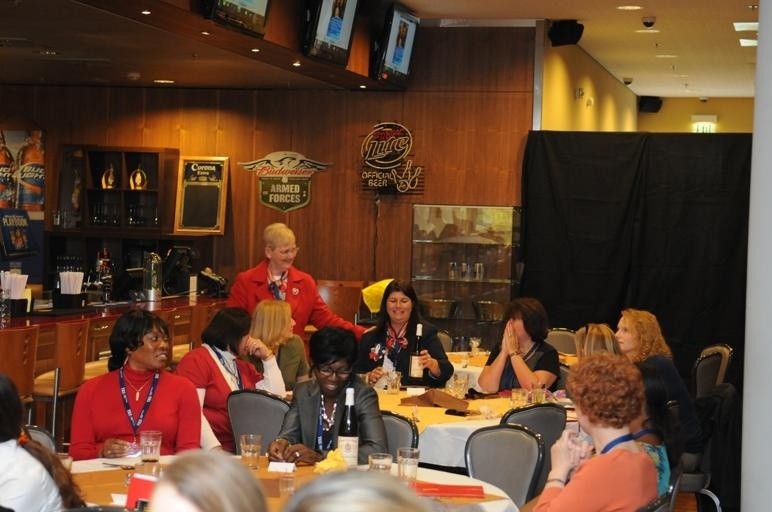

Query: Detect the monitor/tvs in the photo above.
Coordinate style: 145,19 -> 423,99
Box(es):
194,0 -> 270,39
305,0 -> 359,68
371,0 -> 421,90
163,245 -> 192,283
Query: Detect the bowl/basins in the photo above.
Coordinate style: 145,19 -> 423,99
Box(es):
417,299 -> 463,319
472,300 -> 506,320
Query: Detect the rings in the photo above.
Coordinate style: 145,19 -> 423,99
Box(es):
253,342 -> 256,345
295,452 -> 300,457
509,334 -> 512,337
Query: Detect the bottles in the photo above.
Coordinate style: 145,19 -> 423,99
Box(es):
1,133 -> 18,216
70,151 -> 84,219
94,247 -> 114,301
407,322 -> 426,382
15,130 -> 46,212
143,253 -> 163,301
56,255 -> 85,272
337,385 -> 359,470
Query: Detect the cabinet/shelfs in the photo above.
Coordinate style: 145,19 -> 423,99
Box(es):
90,150 -> 161,228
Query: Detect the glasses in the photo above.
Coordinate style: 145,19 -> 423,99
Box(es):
318,366 -> 352,379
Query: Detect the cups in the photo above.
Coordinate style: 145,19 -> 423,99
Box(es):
448,261 -> 485,280
56,454 -> 73,474
386,370 -> 402,394
137,429 -> 163,462
511,389 -> 529,408
368,451 -> 393,473
396,445 -> 420,489
531,380 -> 547,404
238,433 -> 263,469
279,474 -> 298,500
453,371 -> 469,397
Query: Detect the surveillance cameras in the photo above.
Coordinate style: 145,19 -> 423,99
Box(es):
623,78 -> 632,85
642,17 -> 656,29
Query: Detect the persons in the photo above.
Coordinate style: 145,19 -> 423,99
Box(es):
524,307 -> 710,512
265,328 -> 389,468
251,299 -> 311,399
474,298 -> 561,394
171,307 -> 286,452
145,451 -> 264,512
354,280 -> 453,389
282,470 -> 447,512
68,308 -> 201,460
1,373 -> 85,512
226,223 -> 376,359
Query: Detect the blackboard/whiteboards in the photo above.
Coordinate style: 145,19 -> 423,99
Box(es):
173,156 -> 229,236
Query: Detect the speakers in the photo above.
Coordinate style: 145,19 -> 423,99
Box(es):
636,94 -> 663,114
549,19 -> 583,47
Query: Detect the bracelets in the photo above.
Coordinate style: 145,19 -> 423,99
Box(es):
263,350 -> 273,361
365,373 -> 370,383
510,350 -> 521,357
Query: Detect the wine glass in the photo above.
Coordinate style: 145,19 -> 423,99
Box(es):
467,336 -> 482,348
89,201 -> 148,227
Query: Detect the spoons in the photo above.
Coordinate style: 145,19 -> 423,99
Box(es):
101,461 -> 135,470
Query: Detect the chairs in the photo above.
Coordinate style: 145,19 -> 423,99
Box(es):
25,326 -> 733,512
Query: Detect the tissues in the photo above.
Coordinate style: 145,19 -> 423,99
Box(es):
1,269 -> 30,318
52,270 -> 88,310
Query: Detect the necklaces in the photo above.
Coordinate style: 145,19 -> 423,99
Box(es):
122,371 -> 154,402
319,392 -> 338,429
